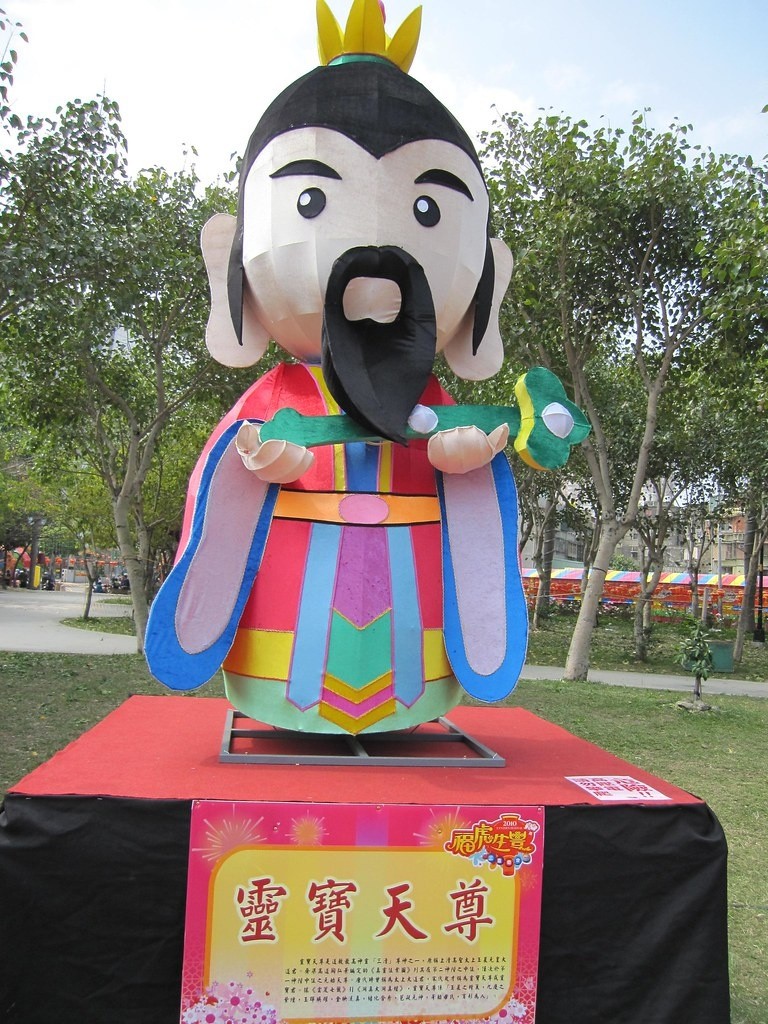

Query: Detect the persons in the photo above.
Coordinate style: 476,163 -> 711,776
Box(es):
6,550 -> 131,596
